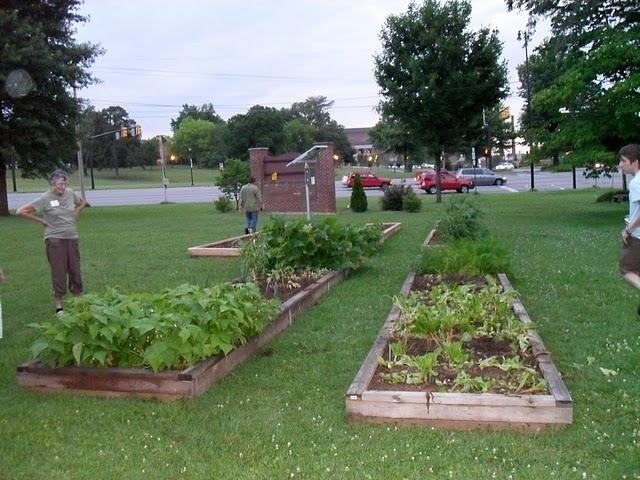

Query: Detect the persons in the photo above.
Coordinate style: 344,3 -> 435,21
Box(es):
618,144 -> 640,316
239,176 -> 264,234
16,170 -> 87,317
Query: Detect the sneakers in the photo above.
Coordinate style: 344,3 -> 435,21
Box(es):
55,307 -> 64,319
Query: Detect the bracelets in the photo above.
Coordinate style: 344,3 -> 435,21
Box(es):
625,227 -> 631,234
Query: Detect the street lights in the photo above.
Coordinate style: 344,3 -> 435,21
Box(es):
516,29 -> 534,190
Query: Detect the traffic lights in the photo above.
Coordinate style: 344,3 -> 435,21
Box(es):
120,125 -> 142,138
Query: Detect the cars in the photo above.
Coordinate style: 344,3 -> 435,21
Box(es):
346,170 -> 393,192
416,162 -> 514,194
